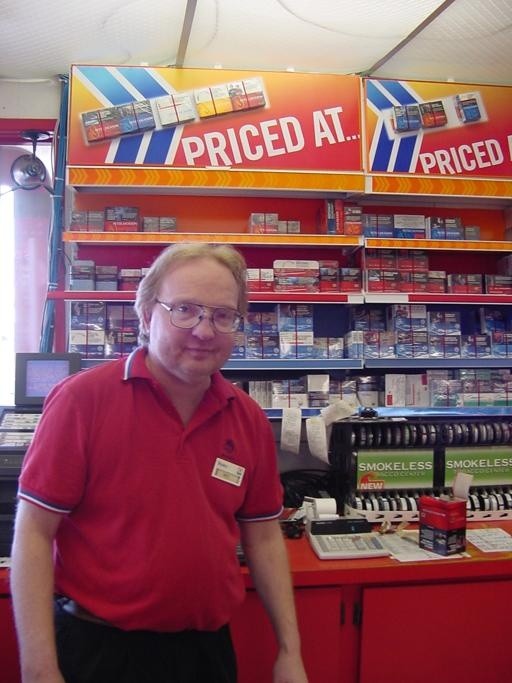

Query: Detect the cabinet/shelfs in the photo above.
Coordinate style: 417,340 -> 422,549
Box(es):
61,195 -> 512,421
2,510 -> 511,682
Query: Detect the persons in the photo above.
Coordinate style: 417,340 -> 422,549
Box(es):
9,240 -> 310,683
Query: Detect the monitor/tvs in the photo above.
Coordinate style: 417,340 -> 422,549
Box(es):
15,353 -> 82,406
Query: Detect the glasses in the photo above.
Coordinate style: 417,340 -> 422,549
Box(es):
155,300 -> 242,333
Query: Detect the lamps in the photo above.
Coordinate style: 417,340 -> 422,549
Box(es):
9,129 -> 47,190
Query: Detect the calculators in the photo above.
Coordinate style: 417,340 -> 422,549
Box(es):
305,516 -> 390,560
